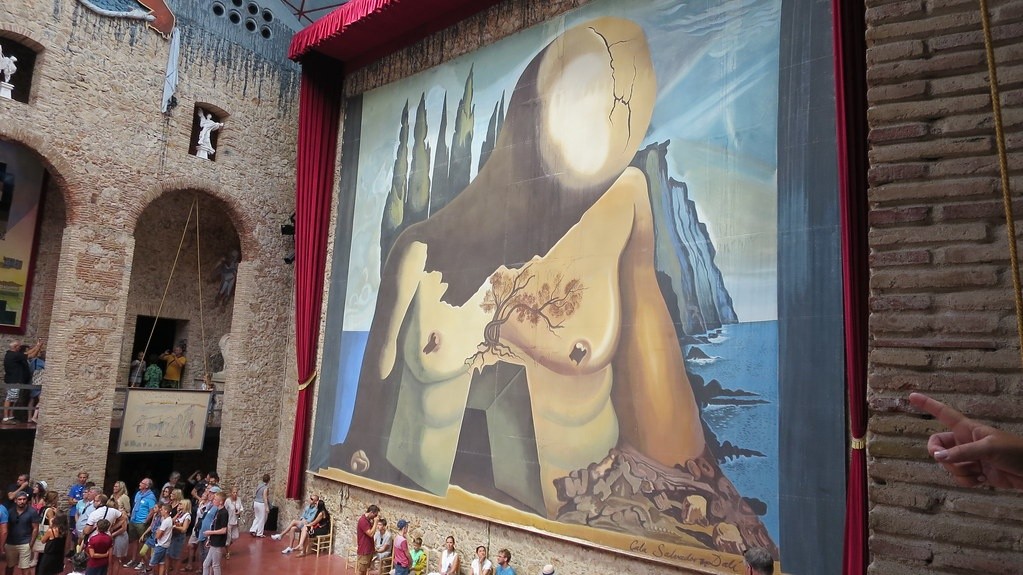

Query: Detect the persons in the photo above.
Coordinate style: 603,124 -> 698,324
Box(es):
291,500 -> 330,558
542,565 -> 557,575
2,341 -> 44,425
201,373 -> 216,422
393,520 -> 413,575
0,468 -> 243,575
372,518 -> 392,559
159,343 -> 187,388
143,354 -> 162,389
488,548 -> 518,575
469,546 -> 493,575
387,537 -> 426,575
270,495 -> 319,553
435,536 -> 459,575
210,249 -> 239,314
249,474 -> 271,537
20,337 -> 42,424
907,393 -> 1023,490
130,351 -> 147,387
355,505 -> 380,575
195,107 -> 222,147
742,547 -> 774,575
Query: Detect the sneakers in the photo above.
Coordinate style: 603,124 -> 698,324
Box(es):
270,534 -> 282,541
282,547 -> 294,553
134,562 -> 145,570
123,560 -> 136,568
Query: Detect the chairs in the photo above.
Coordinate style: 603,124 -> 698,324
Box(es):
297,514 -> 495,575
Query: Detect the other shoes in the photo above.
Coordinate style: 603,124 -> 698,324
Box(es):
66,550 -> 76,558
179,566 -> 194,572
30,559 -> 39,567
295,552 -> 306,558
293,545 -> 303,551
257,533 -> 267,538
250,530 -> 256,536
225,553 -> 230,559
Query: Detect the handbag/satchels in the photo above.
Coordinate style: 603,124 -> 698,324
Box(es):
84,534 -> 89,545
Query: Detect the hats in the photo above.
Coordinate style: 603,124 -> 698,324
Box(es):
36,480 -> 48,490
209,486 -> 222,493
397,519 -> 410,528
542,565 -> 555,575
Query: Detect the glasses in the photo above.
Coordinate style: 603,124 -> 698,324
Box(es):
140,481 -> 148,486
310,498 -> 314,500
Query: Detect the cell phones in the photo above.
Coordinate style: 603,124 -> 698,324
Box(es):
38,338 -> 41,344
